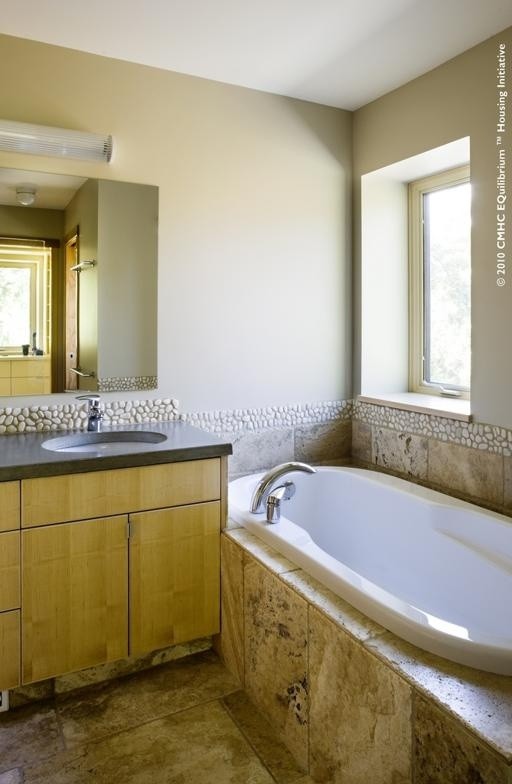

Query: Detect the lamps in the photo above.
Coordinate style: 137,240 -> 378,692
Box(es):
15,188 -> 35,205
0,119 -> 113,163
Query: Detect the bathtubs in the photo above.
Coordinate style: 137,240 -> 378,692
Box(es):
228,466 -> 512,677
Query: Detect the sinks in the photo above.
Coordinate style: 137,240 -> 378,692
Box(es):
40,431 -> 167,452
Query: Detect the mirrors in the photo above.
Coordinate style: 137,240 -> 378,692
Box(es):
0,167 -> 159,397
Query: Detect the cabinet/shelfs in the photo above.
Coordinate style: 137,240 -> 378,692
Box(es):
1,456 -> 229,712
0,360 -> 51,396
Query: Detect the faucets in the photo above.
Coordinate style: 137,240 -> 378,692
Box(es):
75,395 -> 102,430
248,462 -> 317,514
